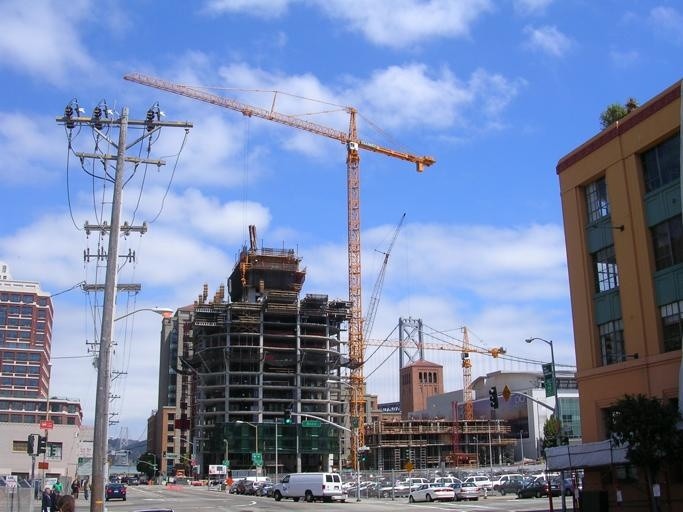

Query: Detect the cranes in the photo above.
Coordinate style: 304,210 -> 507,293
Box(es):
120,68 -> 437,467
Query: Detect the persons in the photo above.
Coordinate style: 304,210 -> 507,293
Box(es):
111,472 -> 126,483
41,477 -> 90,512
625,98 -> 636,113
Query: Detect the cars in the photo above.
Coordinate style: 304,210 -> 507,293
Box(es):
111,473 -> 278,498
337,470 -> 582,504
0,478 -> 43,500
104,483 -> 128,501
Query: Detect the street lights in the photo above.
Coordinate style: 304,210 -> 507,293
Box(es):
324,378 -> 360,503
87,307 -> 174,511
146,452 -> 155,480
523,335 -> 577,494
41,360 -> 53,493
340,325 -> 508,419
170,433 -> 198,447
234,419 -> 258,482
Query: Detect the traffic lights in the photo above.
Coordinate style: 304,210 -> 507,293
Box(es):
136,459 -> 139,464
488,386 -> 497,408
284,409 -> 291,424
37,435 -> 46,455
49,442 -> 56,456
162,450 -> 166,457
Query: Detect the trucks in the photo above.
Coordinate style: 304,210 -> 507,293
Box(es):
265,472 -> 342,503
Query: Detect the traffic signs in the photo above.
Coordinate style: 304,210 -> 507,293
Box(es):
300,420 -> 321,428
